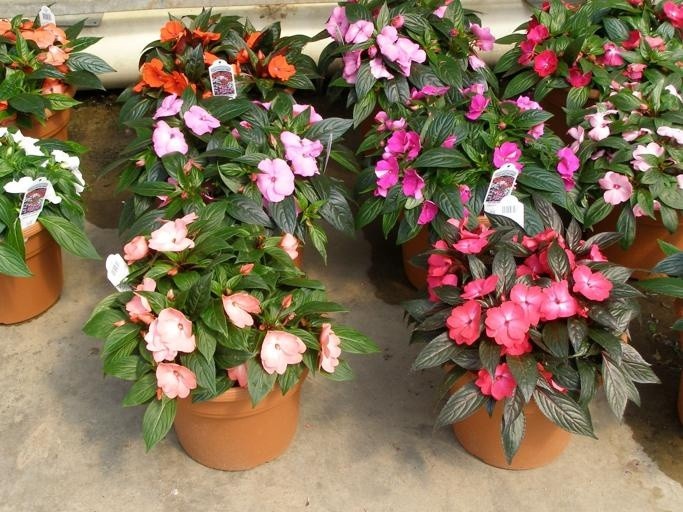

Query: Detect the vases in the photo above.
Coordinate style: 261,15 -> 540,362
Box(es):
176,365 -> 310,472
441,355 -> 576,469
22,104 -> 73,144
0,216 -> 64,327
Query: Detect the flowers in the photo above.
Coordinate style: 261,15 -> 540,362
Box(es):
320,1 -> 681,466
0,1 -> 120,116
0,123 -> 106,275
74,3 -> 385,453
